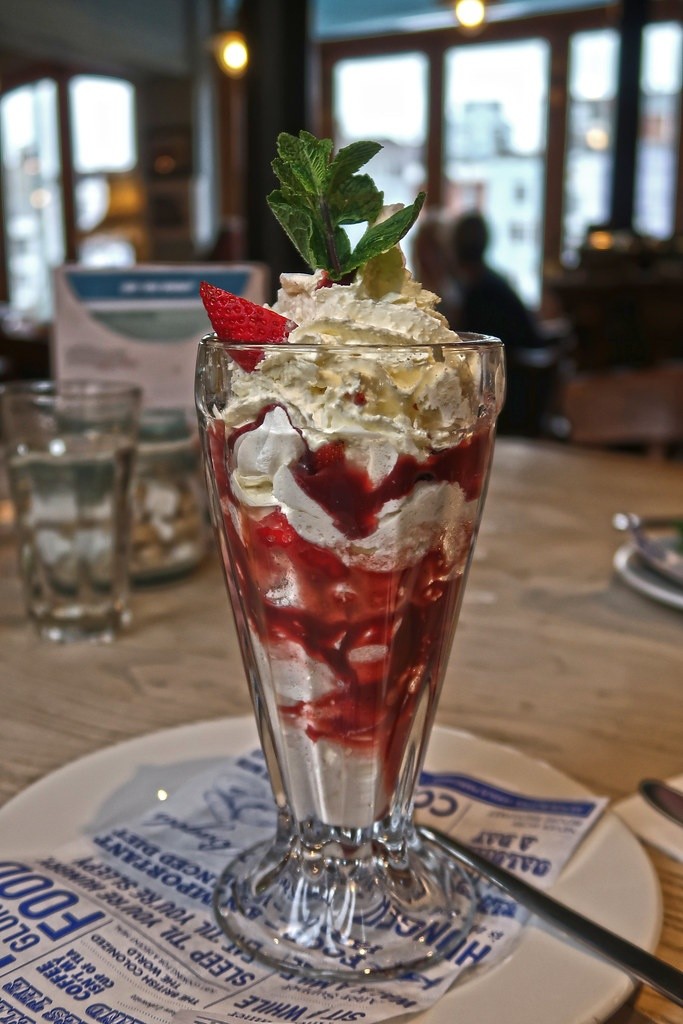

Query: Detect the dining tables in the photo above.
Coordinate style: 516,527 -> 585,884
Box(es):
2,427 -> 683,1024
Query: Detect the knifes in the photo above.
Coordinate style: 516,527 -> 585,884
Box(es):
415,819 -> 683,1008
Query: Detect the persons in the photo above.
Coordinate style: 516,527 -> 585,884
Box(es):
413,216 -> 465,338
447,210 -> 549,434
195,216 -> 246,266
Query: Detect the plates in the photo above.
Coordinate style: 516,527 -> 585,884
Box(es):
613,539 -> 683,614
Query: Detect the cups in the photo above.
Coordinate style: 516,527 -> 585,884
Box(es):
86,403 -> 212,596
3,373 -> 144,645
189,332 -> 506,980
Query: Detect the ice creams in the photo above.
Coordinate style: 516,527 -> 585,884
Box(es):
188,268 -> 495,821
